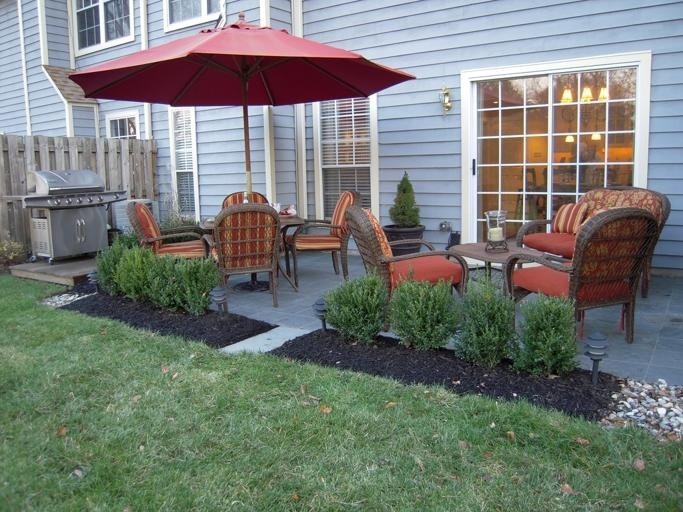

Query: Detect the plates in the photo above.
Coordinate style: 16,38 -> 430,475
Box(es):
279,210 -> 296,218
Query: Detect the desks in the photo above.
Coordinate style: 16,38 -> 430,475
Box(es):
448,243 -> 550,294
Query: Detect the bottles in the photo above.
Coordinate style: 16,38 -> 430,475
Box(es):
243,192 -> 248,204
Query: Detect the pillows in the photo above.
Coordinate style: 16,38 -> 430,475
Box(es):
552,201 -> 588,234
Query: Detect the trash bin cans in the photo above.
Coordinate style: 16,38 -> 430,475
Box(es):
108,228 -> 122,246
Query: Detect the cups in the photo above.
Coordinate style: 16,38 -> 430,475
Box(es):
272,202 -> 280,213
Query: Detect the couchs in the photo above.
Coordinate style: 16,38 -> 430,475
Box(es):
515,184 -> 670,297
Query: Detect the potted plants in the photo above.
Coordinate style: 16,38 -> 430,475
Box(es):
382,169 -> 426,256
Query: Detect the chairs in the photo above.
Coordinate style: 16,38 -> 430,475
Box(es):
501,208 -> 661,345
342,207 -> 471,308
125,190 -> 360,313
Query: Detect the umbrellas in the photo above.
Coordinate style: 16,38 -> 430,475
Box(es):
63,11 -> 419,283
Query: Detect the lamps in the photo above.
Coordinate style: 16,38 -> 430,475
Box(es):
560,86 -> 610,103
440,85 -> 452,112
564,134 -> 575,143
591,133 -> 602,141
